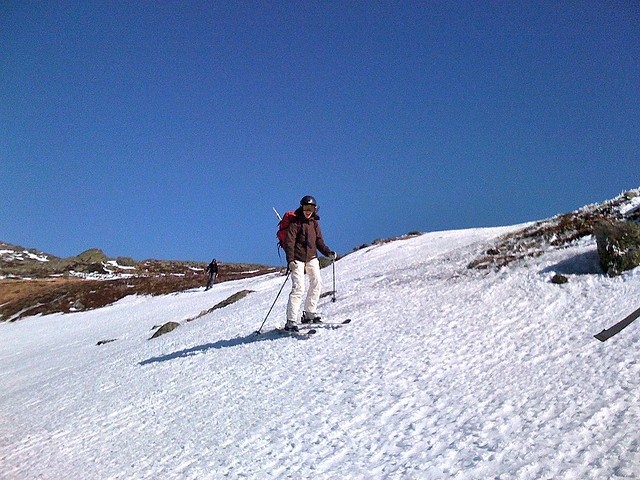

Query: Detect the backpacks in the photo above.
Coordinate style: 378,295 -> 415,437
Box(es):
276,211 -> 299,259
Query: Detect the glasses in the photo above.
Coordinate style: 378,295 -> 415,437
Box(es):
302,204 -> 319,212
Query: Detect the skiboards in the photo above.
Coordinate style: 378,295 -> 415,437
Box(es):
274,319 -> 351,335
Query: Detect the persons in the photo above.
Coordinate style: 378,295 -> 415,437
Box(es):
285,196 -> 337,331
204,259 -> 219,290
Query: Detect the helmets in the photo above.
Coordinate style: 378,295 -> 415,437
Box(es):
300,195 -> 316,206
212,259 -> 217,262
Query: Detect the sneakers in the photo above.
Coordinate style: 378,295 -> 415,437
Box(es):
300,312 -> 322,323
284,321 -> 300,333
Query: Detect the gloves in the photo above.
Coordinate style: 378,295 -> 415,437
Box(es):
329,251 -> 337,259
288,261 -> 298,270
